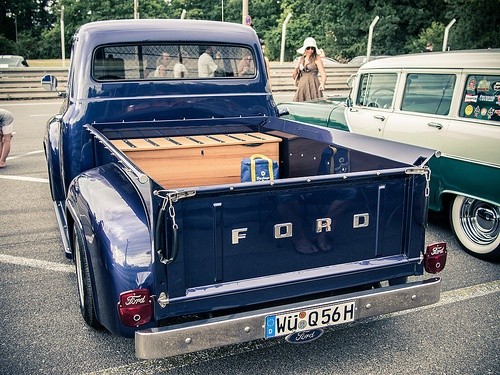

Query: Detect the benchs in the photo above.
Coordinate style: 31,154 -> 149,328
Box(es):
405,94 -> 454,113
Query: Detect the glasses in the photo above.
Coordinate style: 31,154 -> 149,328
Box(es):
306,47 -> 315,50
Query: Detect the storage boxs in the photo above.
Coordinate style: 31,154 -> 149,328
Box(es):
109,132 -> 281,189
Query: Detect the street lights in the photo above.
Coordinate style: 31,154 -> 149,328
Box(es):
5,12 -> 17,42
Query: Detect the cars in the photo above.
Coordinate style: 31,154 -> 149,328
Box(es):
0,55 -> 28,68
275,48 -> 500,264
293,56 -> 340,64
348,55 -> 390,66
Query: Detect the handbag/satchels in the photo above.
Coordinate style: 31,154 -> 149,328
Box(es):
294,57 -> 302,87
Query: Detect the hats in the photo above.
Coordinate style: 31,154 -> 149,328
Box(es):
296,37 -> 323,56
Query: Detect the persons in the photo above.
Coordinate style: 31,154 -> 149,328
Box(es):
198,47 -> 221,78
237,39 -> 271,93
293,36 -> 327,102
423,43 -> 433,53
174,50 -> 189,78
0,108 -> 15,166
154,53 -> 171,78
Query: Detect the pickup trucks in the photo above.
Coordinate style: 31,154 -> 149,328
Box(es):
41,19 -> 447,362
